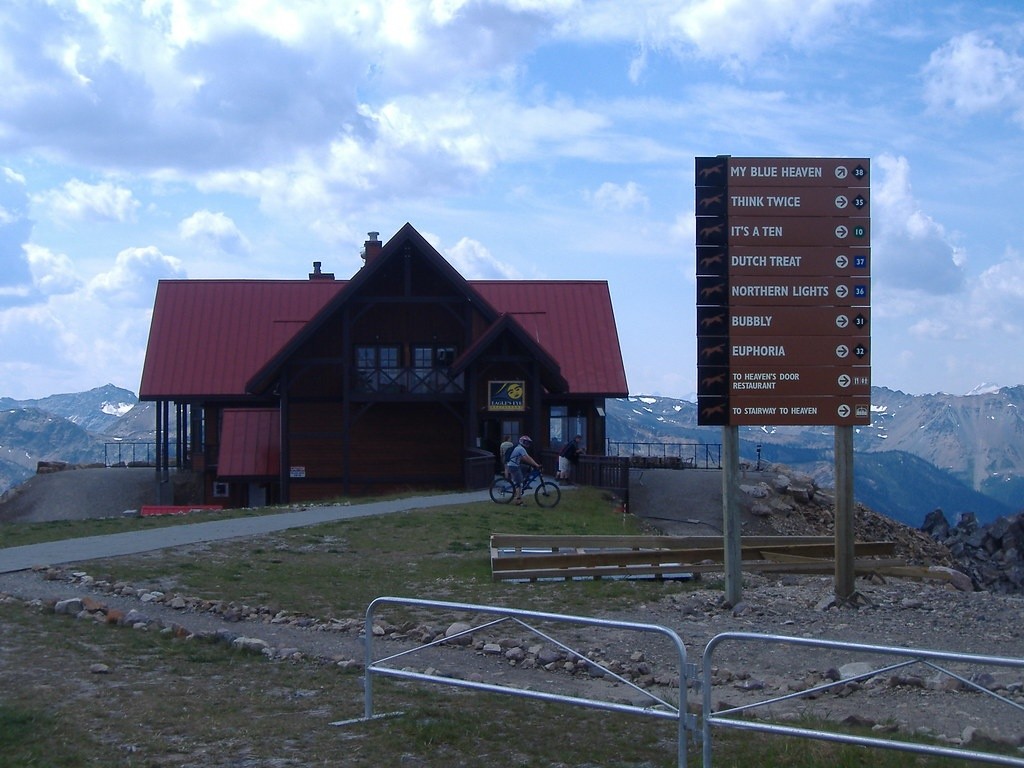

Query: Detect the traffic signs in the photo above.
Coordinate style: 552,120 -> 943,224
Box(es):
694,154 -> 872,426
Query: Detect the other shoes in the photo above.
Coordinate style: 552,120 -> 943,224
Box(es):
526,486 -> 532,489
558,479 -> 572,485
517,501 -> 526,507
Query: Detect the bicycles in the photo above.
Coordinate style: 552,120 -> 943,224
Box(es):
489,461 -> 562,509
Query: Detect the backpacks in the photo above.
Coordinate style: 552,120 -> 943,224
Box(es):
504,443 -> 526,464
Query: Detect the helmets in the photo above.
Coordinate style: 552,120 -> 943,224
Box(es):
519,436 -> 532,448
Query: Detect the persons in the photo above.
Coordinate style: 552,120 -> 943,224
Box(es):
507,435 -> 542,507
500,435 -> 515,482
555,435 -> 585,486
